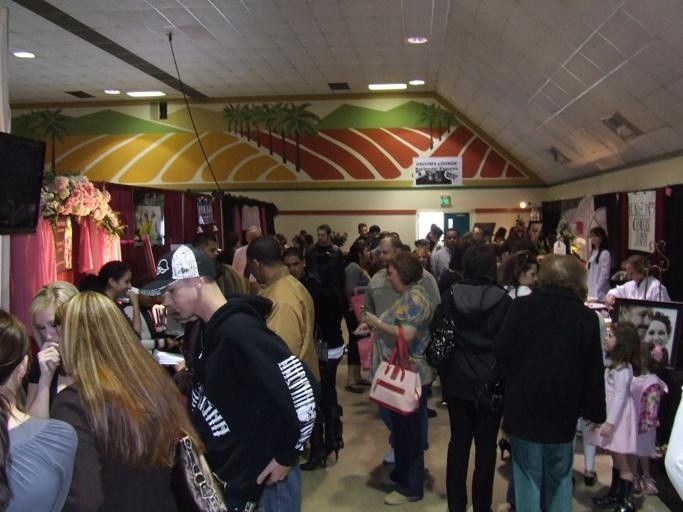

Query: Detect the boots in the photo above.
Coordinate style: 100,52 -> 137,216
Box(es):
592,467 -> 634,512
300,411 -> 327,471
324,405 -> 344,461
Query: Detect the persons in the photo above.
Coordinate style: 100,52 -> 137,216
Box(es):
1,221 -> 682,511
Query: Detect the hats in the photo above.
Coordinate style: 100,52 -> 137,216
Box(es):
138,243 -> 218,296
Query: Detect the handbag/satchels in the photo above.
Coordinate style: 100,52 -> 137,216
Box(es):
171,425 -> 260,511
368,324 -> 424,417
424,293 -> 457,366
312,327 -> 331,363
357,335 -> 374,371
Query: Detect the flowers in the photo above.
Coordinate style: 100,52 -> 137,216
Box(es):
40,174 -> 122,237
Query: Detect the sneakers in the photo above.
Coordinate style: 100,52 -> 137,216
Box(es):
383,447 -> 394,462
426,407 -> 438,417
385,489 -> 421,506
346,378 -> 370,394
390,469 -> 428,483
633,473 -> 660,494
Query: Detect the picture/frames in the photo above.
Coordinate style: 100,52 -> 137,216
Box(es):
605,298 -> 683,371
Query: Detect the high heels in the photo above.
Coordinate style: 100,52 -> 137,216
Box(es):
498,437 -> 512,461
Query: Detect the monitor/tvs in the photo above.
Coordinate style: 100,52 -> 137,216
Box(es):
0,132 -> 46,234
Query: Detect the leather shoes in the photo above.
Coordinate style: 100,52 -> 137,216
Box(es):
584,470 -> 597,486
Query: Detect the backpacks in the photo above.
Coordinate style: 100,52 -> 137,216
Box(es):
443,336 -> 504,414
